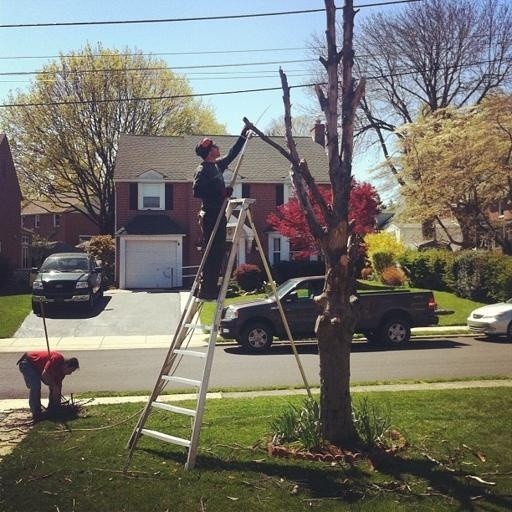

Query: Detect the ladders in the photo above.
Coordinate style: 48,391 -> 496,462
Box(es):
126,198 -> 313,472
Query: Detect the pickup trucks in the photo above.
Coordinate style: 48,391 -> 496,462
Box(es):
219,275 -> 439,348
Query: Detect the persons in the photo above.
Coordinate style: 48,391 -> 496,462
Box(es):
192,122 -> 253,301
18,352 -> 80,423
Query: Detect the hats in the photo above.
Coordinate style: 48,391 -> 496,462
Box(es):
195,136 -> 213,156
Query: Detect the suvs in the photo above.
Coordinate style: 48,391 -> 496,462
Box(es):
31,252 -> 107,315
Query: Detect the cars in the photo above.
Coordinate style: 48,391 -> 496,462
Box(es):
465,295 -> 511,340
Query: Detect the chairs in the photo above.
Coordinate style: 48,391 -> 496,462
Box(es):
47,260 -> 88,269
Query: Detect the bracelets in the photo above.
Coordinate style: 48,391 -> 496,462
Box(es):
240,134 -> 246,140
